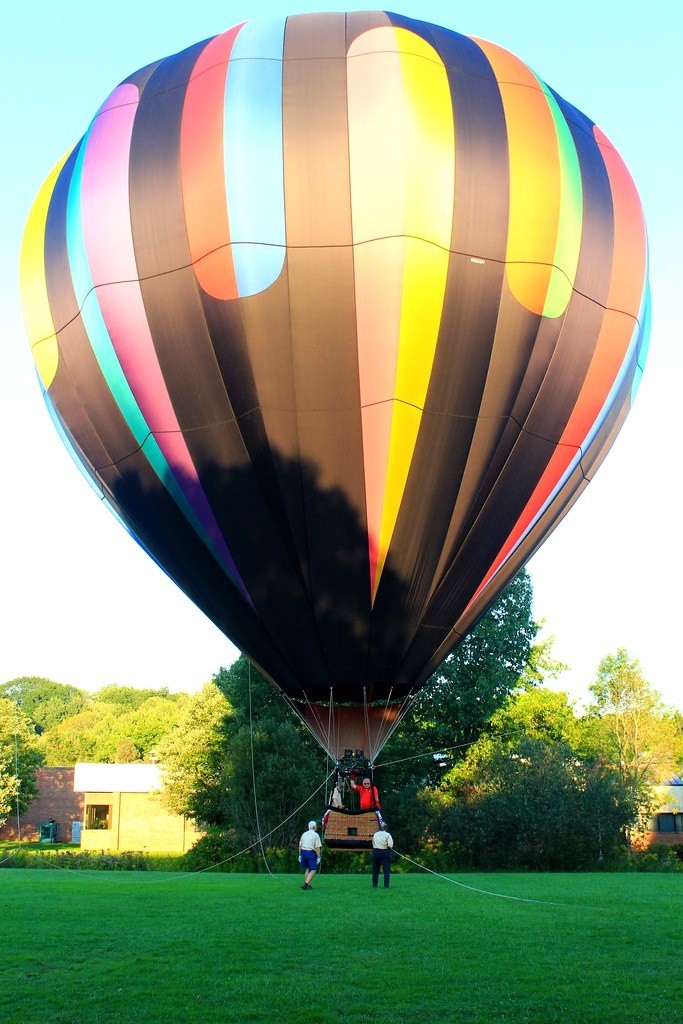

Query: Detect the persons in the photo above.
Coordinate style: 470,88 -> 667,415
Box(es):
342,777 -> 382,812
329,783 -> 348,812
297,821 -> 323,892
371,821 -> 393,888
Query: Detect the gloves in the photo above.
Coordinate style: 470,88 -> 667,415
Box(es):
298,855 -> 302,862
316,857 -> 321,865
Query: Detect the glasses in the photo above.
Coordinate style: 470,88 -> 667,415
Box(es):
364,783 -> 369,785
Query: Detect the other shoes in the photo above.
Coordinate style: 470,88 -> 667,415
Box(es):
308,886 -> 312,889
301,884 -> 308,890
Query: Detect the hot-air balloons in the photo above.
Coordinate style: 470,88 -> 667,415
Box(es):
15,10 -> 652,852
